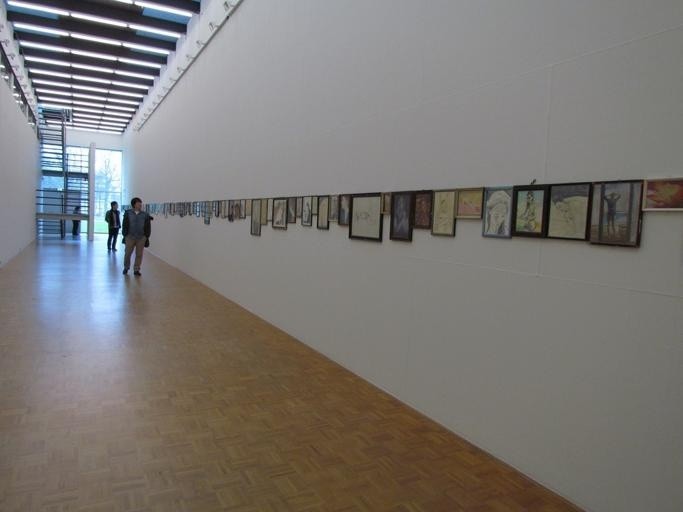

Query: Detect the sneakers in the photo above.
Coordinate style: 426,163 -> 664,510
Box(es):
134,271 -> 141,276
123,267 -> 129,274
108,248 -> 117,251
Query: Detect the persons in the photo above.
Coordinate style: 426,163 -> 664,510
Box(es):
604,192 -> 621,241
123,198 -> 153,275
105,201 -> 121,251
553,196 -> 588,219
519,191 -> 537,231
72,207 -> 80,235
341,196 -> 349,224
487,190 -> 511,235
393,195 -> 409,234
417,199 -> 430,226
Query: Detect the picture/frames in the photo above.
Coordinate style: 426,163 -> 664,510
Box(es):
511,183 -> 549,238
389,191 -> 416,242
412,190 -> 434,230
455,187 -> 485,219
545,181 -> 590,240
121,189 -> 349,236
380,193 -> 392,214
588,179 -> 644,248
348,192 -> 382,243
482,185 -> 512,240
431,189 -> 456,237
641,176 -> 682,211
